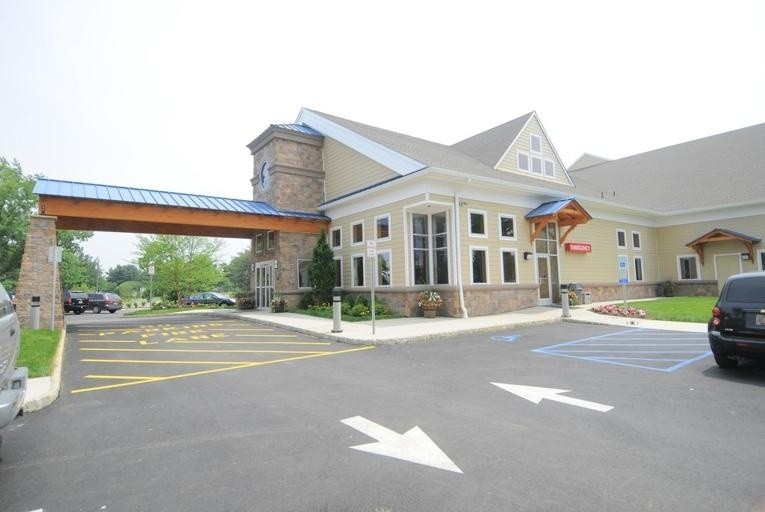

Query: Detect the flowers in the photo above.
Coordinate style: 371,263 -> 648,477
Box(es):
417,289 -> 443,307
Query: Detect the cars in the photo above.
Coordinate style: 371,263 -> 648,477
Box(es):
186,291 -> 236,308
87,293 -> 123,314
708,271 -> 765,369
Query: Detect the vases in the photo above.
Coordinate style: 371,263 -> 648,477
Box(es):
422,306 -> 437,318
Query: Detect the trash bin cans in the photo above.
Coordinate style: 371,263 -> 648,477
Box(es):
568,283 -> 591,305
657,281 -> 673,297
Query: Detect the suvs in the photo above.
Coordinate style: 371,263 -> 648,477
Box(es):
64,290 -> 90,315
0,282 -> 29,428
